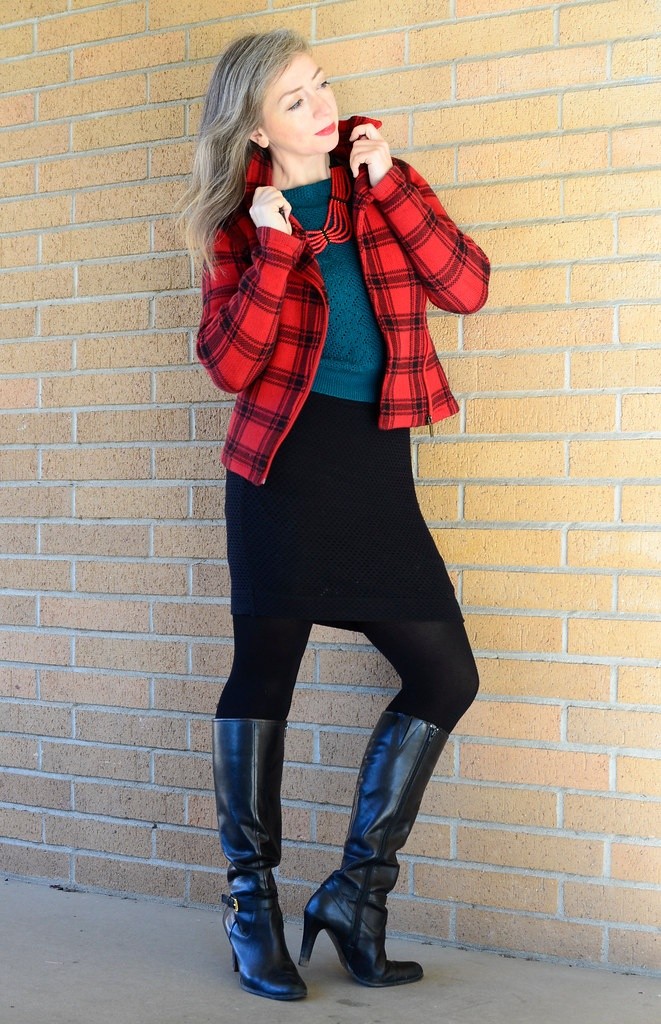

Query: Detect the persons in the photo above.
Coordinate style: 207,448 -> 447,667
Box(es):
172,26 -> 493,999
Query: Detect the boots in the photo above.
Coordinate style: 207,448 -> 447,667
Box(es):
213,717 -> 310,1001
297,712 -> 450,989
